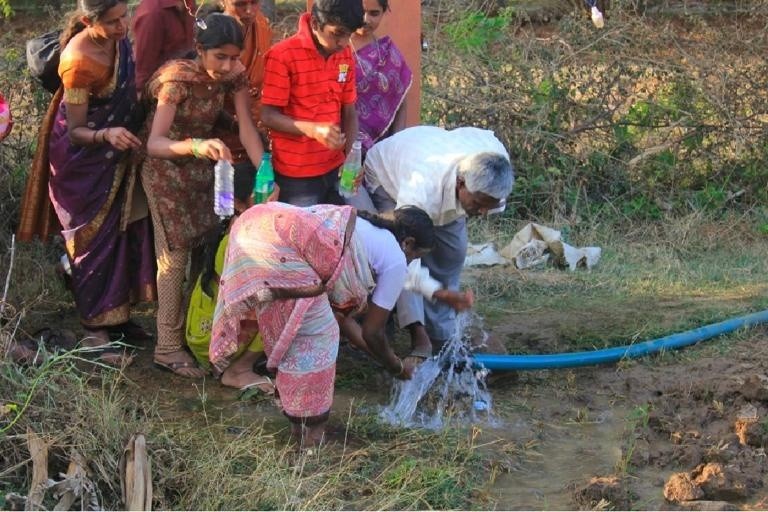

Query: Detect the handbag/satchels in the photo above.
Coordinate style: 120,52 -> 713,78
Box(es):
25,28 -> 63,95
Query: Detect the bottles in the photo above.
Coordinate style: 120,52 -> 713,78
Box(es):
339,140 -> 362,199
213,157 -> 235,217
254,153 -> 276,204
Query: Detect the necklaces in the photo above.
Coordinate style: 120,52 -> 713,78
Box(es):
349,32 -> 389,81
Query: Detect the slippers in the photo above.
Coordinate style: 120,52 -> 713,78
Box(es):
221,371 -> 278,395
18,319 -> 76,351
78,344 -> 134,366
153,350 -> 211,380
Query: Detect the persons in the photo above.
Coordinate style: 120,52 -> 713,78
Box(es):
362,125 -> 515,352
15,0 -> 157,369
259,1 -> 358,208
350,0 -> 414,156
129,0 -> 201,106
218,0 -> 275,161
206,199 -> 438,452
127,12 -> 279,379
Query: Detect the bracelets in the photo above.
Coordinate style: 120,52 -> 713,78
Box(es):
392,358 -> 408,377
93,127 -> 108,143
187,136 -> 203,159
